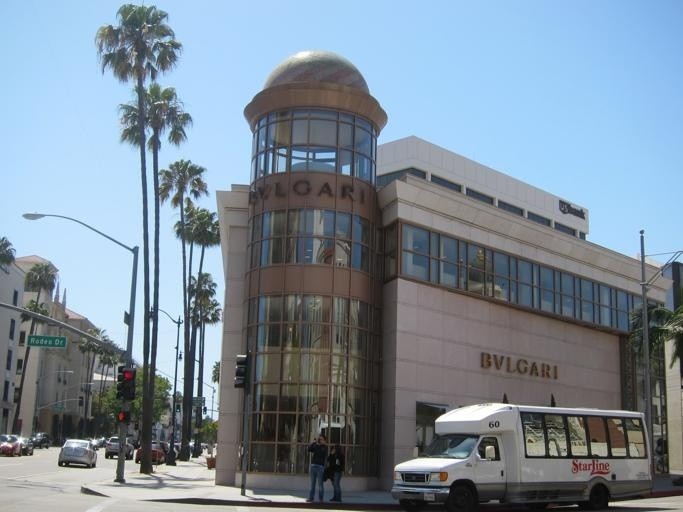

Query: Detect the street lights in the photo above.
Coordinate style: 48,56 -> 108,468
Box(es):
148,307 -> 215,449
22,214 -> 138,479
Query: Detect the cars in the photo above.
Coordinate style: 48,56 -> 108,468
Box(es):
106,436 -> 134,460
136,441 -> 209,465
1,431 -> 53,457
58,436 -> 110,468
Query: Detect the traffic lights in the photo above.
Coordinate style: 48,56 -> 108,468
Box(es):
116,363 -> 136,402
119,411 -> 130,426
234,350 -> 247,388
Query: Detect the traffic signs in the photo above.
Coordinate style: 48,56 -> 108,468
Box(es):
27,335 -> 68,348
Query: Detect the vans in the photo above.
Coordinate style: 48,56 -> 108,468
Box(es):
391,401 -> 654,510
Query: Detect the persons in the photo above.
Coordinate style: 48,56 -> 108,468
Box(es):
326,443 -> 345,502
305,434 -> 328,502
